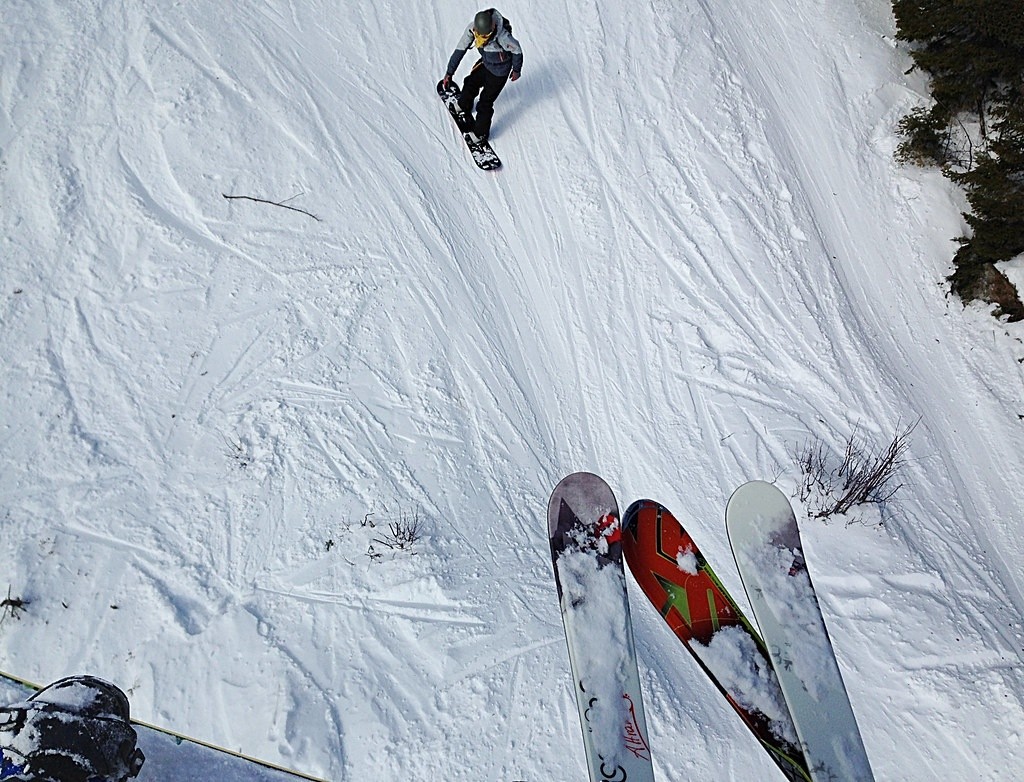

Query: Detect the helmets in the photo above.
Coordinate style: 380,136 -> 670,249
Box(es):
474,11 -> 493,34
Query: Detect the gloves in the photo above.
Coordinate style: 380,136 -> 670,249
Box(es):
509,69 -> 521,82
443,71 -> 452,90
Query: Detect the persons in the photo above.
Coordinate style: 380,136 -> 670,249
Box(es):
444,8 -> 523,147
0,674 -> 130,782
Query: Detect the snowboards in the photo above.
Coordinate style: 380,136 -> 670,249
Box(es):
2,672 -> 320,781
542,469 -> 878,781
436,78 -> 502,171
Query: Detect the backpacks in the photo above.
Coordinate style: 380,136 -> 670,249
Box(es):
502,16 -> 512,38
